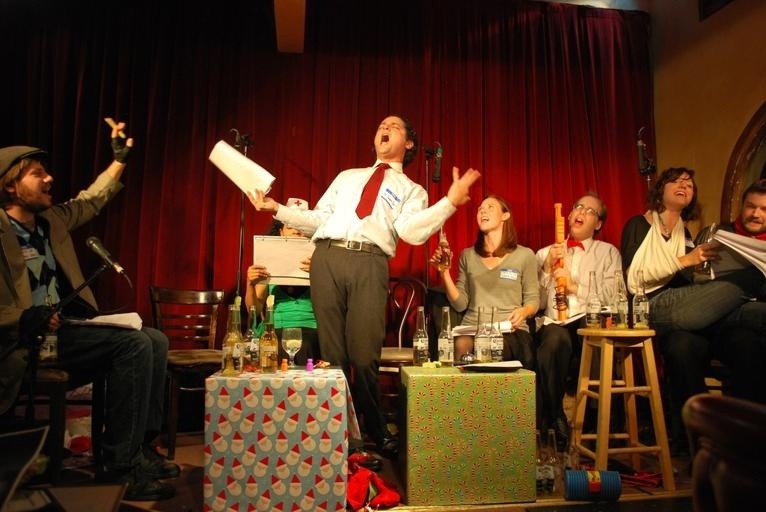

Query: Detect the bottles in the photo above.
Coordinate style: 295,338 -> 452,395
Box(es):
693,221 -> 717,275
475,305 -> 492,362
221,294 -> 278,378
412,306 -> 429,367
281,359 -> 288,374
535,424 -> 580,496
437,306 -> 454,367
437,232 -> 451,271
631,268 -> 651,329
611,270 -> 628,330
306,359 -> 313,371
461,351 -> 475,365
585,270 -> 601,328
36,295 -> 59,363
489,305 -> 504,361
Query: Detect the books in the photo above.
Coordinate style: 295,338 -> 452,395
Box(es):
251,235 -> 317,287
708,228 -> 766,281
207,139 -> 277,201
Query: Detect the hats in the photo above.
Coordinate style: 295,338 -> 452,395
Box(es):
0,146 -> 47,177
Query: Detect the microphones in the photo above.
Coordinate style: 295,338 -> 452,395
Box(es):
636,139 -> 648,170
432,146 -> 443,183
85,235 -> 125,275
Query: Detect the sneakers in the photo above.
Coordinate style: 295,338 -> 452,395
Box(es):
93,443 -> 182,500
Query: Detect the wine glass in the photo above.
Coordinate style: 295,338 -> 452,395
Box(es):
281,327 -> 303,371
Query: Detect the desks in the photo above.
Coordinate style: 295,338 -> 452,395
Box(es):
203,366 -> 349,512
401,367 -> 537,507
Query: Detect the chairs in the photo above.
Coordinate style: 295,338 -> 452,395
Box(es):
148,285 -> 225,460
378,276 -> 430,410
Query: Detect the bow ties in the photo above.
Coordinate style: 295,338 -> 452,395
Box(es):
565,239 -> 587,251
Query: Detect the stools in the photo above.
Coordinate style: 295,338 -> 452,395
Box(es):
3,382 -> 76,447
568,328 -> 676,493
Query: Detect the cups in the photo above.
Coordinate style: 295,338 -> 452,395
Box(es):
596,311 -> 621,329
563,470 -> 622,501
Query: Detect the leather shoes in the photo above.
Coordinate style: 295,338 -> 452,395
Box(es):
348,448 -> 384,471
375,426 -> 406,464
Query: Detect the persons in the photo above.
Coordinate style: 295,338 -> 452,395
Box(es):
535,191 -> 631,456
246,115 -> 482,450
0,115 -> 182,501
618,165 -> 766,334
428,195 -> 540,364
729,177 -> 766,243
244,196 -> 321,365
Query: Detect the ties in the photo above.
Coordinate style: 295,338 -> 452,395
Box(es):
355,163 -> 391,220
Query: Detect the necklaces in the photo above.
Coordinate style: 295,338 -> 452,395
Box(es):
658,213 -> 671,238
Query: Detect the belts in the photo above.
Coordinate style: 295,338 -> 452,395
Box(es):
317,239 -> 384,255
